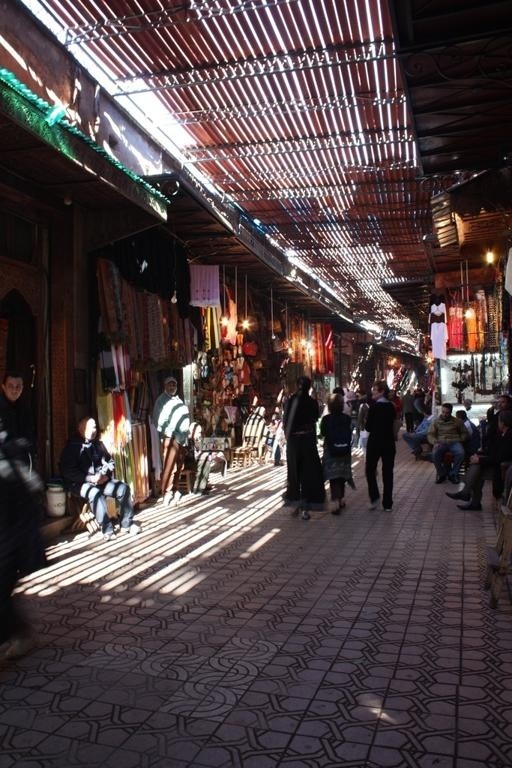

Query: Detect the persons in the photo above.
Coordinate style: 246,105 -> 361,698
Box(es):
282,373 -> 512,521
153,375 -> 191,438
63,417 -> 141,541
182,422 -> 203,471
1,373 -> 48,475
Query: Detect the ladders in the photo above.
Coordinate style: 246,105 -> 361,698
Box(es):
484,490 -> 512,609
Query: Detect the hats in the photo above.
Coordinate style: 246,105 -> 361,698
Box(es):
164,376 -> 178,385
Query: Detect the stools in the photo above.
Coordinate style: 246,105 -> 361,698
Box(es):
231,446 -> 255,468
448,452 -> 470,480
172,470 -> 196,496
61,497 -> 101,538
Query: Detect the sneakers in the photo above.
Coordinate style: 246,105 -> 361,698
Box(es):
436,474 -> 447,484
368,499 -> 379,509
103,531 -> 117,540
330,501 -> 347,516
289,505 -> 311,521
449,475 -> 460,484
120,523 -> 140,535
382,504 -> 393,512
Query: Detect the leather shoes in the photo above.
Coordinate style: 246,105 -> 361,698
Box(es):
455,503 -> 483,511
443,490 -> 472,502
